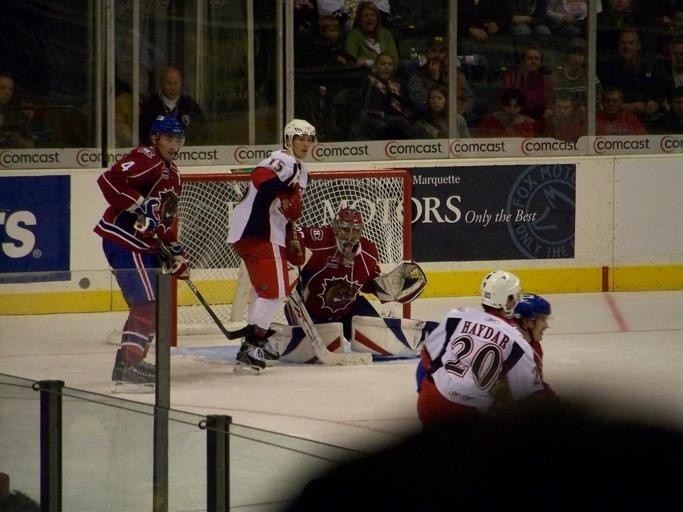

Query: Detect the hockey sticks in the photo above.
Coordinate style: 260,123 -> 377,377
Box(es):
154,234 -> 255,340
289,290 -> 374,365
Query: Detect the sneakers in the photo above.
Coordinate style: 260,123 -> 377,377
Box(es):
260,346 -> 279,360
236,343 -> 265,369
137,360 -> 156,373
112,349 -> 156,383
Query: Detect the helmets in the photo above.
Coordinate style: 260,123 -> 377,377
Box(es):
284,119 -> 316,153
333,208 -> 364,244
481,271 -> 522,319
515,293 -> 551,319
150,116 -> 185,140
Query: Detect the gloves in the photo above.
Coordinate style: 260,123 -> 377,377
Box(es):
286,239 -> 305,265
133,201 -> 157,237
169,245 -> 190,280
279,192 -> 301,221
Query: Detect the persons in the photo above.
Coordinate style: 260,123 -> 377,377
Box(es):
279,206 -> 440,365
223,118 -> 313,379
1,2 -> 680,149
414,291 -> 550,393
417,268 -> 540,430
93,111 -> 184,395
